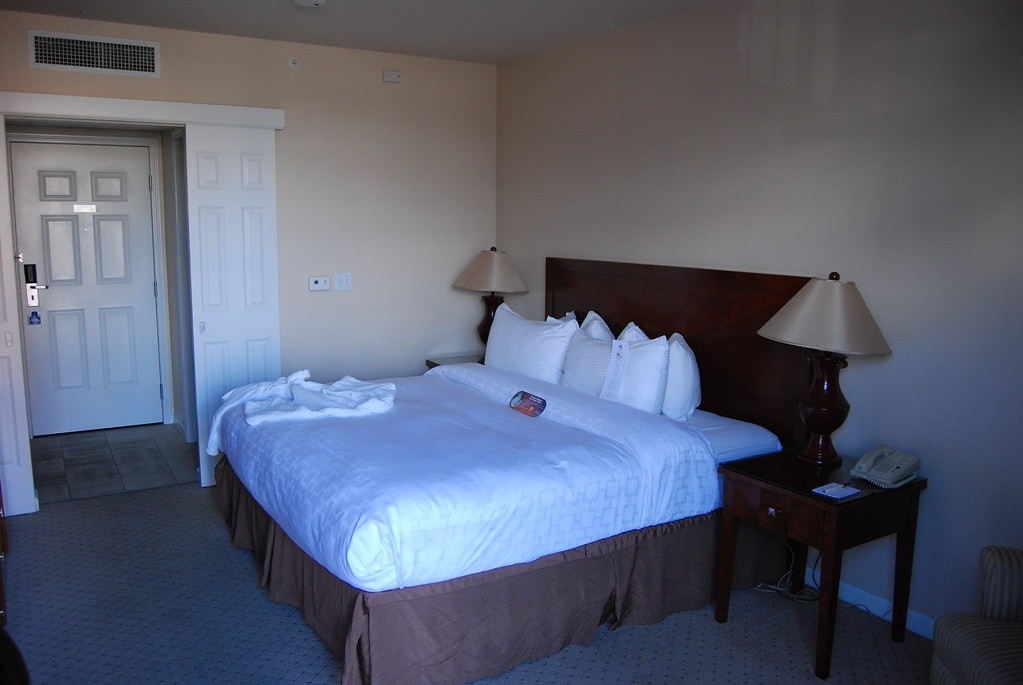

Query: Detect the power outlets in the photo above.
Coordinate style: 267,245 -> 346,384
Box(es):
334,273 -> 353,291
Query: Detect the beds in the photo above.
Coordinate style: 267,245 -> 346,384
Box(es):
207,257 -> 812,685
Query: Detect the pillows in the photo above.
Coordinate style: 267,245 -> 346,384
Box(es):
579,310 -> 615,344
617,321 -> 702,423
483,302 -> 579,385
561,332 -> 669,416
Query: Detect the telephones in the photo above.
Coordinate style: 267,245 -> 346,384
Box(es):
849,445 -> 921,489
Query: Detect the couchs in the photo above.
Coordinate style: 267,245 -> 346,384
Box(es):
930,545 -> 1023,685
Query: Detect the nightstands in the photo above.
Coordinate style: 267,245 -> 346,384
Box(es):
715,445 -> 929,680
425,354 -> 484,370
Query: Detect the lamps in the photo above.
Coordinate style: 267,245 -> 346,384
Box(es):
452,246 -> 529,365
756,272 -> 894,467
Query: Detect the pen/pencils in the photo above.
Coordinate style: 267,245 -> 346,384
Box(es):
823,482 -> 851,492
